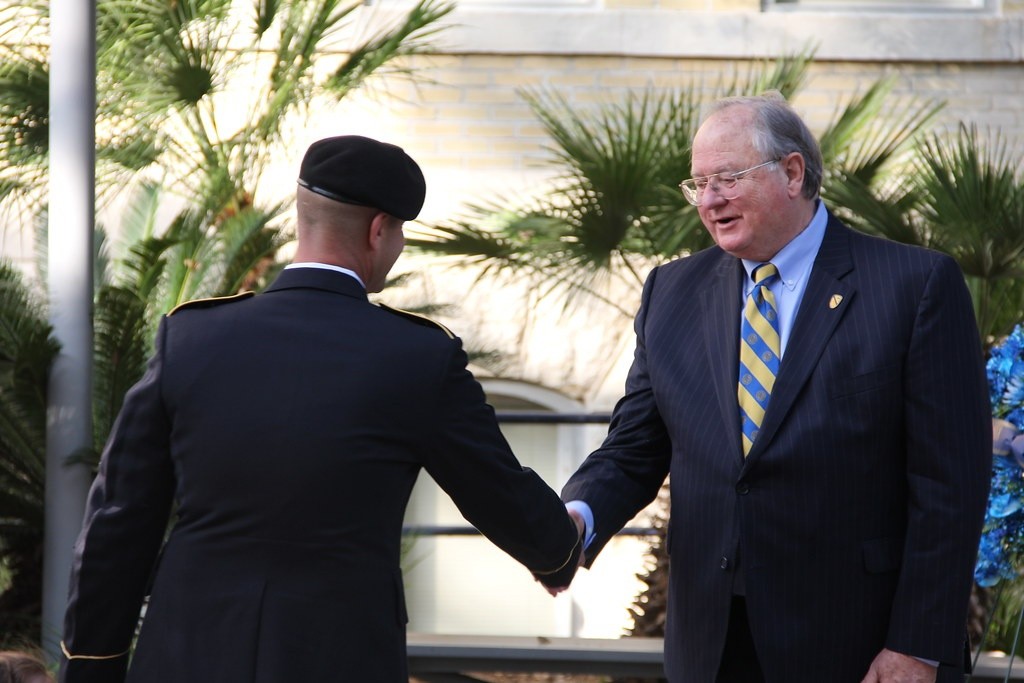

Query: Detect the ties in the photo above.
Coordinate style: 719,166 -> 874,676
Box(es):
738,263 -> 781,462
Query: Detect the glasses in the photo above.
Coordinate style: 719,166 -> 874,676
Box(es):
678,158 -> 782,207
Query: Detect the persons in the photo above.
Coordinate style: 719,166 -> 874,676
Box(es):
555,93 -> 993,683
57,135 -> 577,683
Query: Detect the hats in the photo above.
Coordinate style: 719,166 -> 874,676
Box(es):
298,135 -> 426,221
